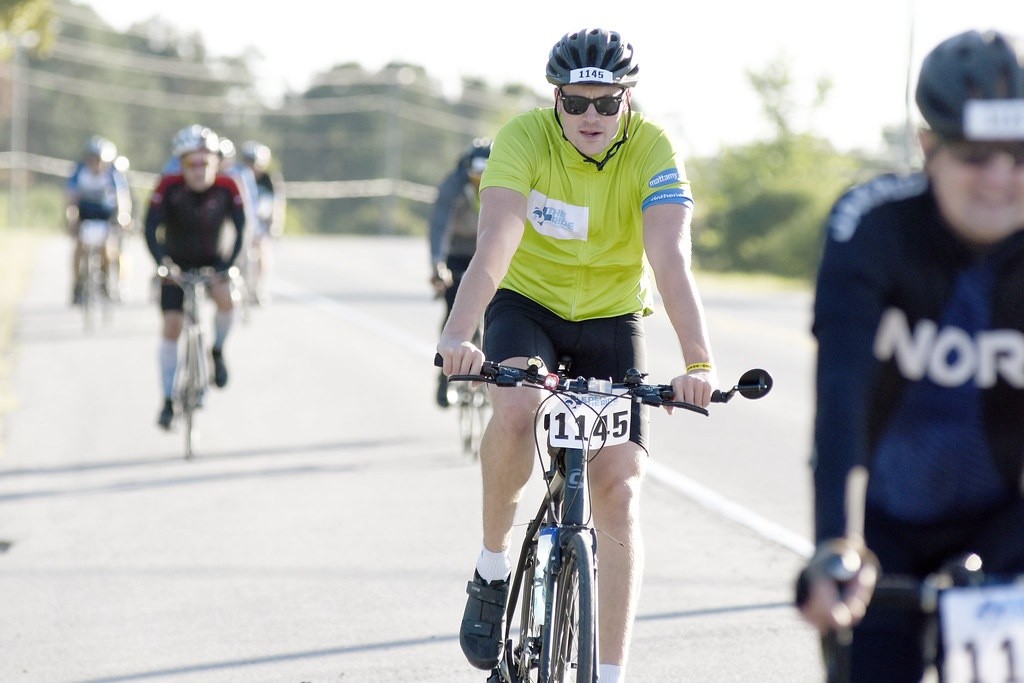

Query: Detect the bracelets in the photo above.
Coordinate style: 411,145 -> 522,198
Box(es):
687,363 -> 711,372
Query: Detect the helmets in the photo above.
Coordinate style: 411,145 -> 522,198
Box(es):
243,141 -> 272,165
545,28 -> 639,88
171,124 -> 219,159
83,137 -> 116,162
468,138 -> 492,176
916,30 -> 1024,142
217,137 -> 235,159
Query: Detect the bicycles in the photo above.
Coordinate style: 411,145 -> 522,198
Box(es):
67,214 -> 134,331
827,546 -> 1023,683
433,352 -> 774,683
153,265 -> 237,462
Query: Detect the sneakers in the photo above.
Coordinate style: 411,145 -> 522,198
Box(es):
459,567 -> 511,670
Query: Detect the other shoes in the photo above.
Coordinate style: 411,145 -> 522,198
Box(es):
159,405 -> 174,429
212,346 -> 228,387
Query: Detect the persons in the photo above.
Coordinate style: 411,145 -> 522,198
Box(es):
144,130 -> 246,428
221,143 -> 276,310
437,27 -> 715,682
66,142 -> 136,306
796,31 -> 1024,683
429,147 -> 492,406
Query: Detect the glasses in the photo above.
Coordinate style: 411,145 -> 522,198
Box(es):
932,125 -> 1024,164
557,87 -> 627,116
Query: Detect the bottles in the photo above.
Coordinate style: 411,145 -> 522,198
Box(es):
533,527 -> 557,625
